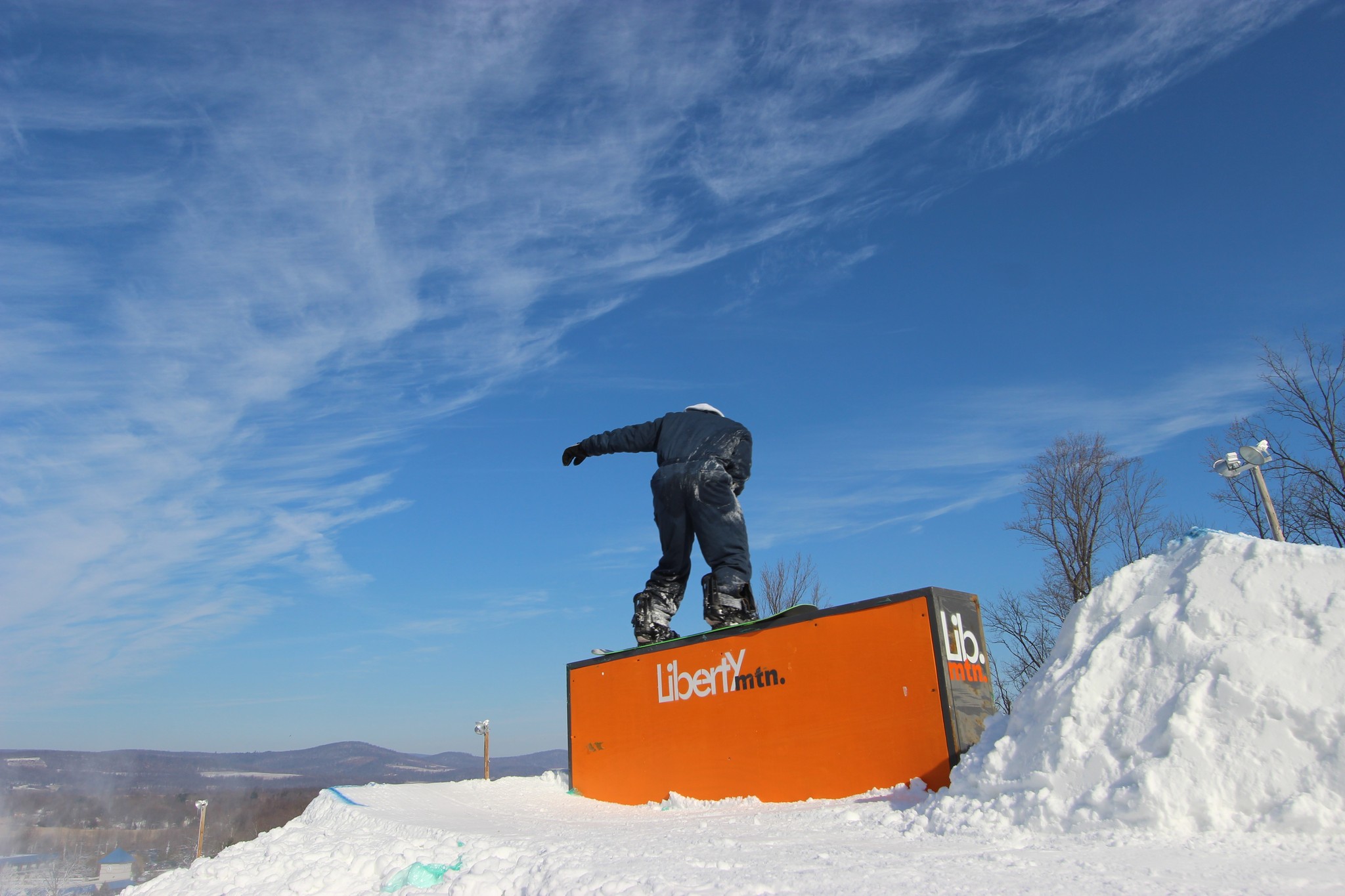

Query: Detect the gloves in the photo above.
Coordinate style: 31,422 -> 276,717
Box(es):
562,443 -> 587,466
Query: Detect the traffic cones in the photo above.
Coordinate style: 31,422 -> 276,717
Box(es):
195,799 -> 208,857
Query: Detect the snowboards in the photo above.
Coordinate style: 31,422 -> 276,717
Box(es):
589,605 -> 818,658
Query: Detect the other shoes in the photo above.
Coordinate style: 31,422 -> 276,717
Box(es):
633,621 -> 678,643
703,604 -> 759,628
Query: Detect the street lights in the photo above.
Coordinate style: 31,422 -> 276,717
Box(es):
1212,439 -> 1287,544
474,719 -> 491,780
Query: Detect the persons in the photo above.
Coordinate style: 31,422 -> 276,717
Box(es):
562,403 -> 757,645
